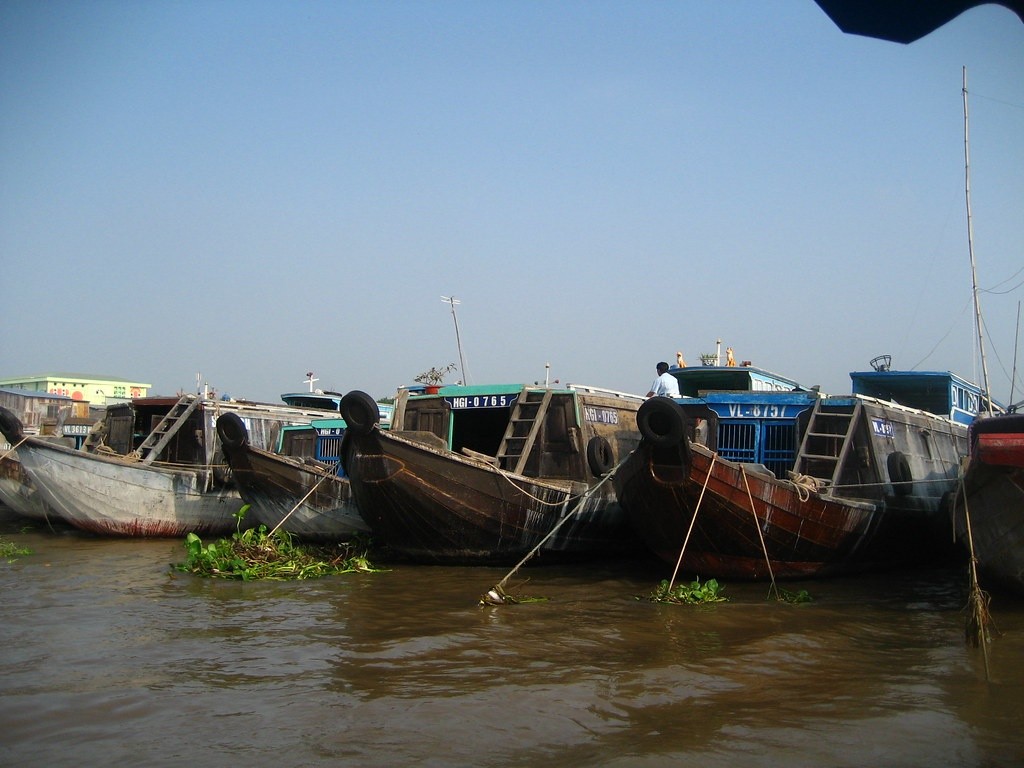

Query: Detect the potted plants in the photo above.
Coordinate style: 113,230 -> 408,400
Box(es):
697,353 -> 721,367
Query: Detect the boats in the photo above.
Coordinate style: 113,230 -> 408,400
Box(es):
214,409 -> 388,541
947,405 -> 1024,590
0,398 -> 392,537
0,417 -> 148,524
377,381 -> 651,556
638,365 -> 1007,582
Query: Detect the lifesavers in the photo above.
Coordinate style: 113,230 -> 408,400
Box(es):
886,451 -> 912,495
587,437 -> 615,480
0,406 -> 24,443
339,389 -> 379,434
636,398 -> 689,450
215,412 -> 248,448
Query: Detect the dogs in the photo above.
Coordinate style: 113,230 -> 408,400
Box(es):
676,351 -> 686,368
724,346 -> 737,367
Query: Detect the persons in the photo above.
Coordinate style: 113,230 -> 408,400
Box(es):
646,362 -> 680,398
